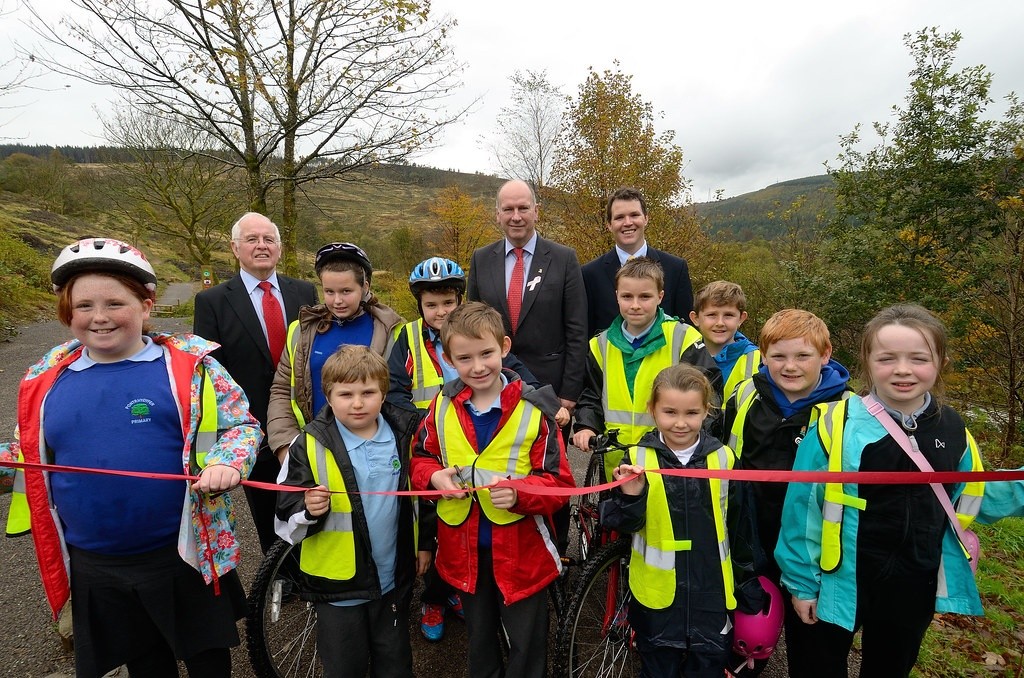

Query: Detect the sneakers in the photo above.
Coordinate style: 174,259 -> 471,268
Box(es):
421,602 -> 444,640
446,591 -> 465,620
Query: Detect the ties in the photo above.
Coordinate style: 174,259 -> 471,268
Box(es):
628,256 -> 634,262
507,248 -> 524,337
257,281 -> 286,372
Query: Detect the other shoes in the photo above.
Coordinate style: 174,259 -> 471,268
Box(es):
269,579 -> 296,602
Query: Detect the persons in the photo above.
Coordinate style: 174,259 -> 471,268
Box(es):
467,179 -> 589,449
267,242 -> 411,466
573,255 -> 703,495
0,238 -> 266,678
192,210 -> 320,602
775,305 -> 1024,678
274,344 -> 437,678
726,308 -> 854,678
690,280 -> 762,413
380,257 -> 571,639
598,362 -> 773,678
582,187 -> 694,351
409,301 -> 577,678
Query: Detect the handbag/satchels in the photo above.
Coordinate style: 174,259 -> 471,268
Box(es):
964,528 -> 979,575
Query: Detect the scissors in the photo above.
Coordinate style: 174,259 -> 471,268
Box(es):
454,465 -> 476,501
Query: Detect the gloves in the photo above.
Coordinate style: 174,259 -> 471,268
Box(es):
732,576 -> 770,616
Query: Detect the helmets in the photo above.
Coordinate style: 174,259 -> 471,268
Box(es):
315,242 -> 373,278
732,576 -> 785,659
51,238 -> 158,302
409,257 -> 466,300
